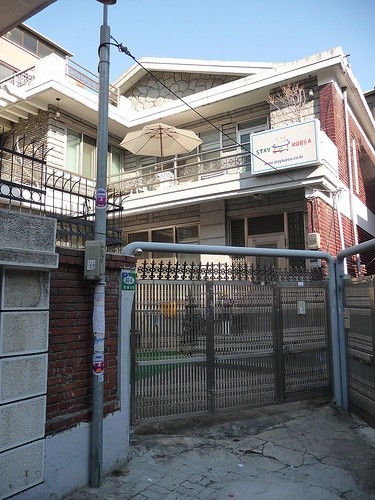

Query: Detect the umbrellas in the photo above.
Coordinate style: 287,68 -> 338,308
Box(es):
120,123 -> 204,164
